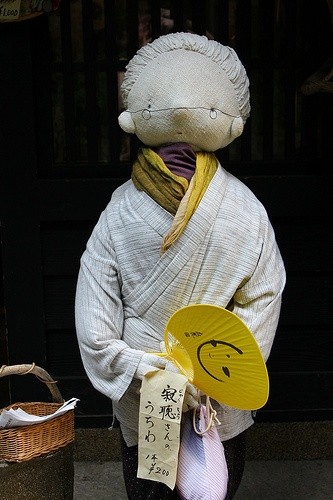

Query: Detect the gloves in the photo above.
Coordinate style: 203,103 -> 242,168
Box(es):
135,342 -> 205,413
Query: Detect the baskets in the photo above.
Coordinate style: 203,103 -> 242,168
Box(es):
0,363 -> 74,463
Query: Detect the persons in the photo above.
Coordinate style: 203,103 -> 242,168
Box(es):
74,32 -> 287,500
